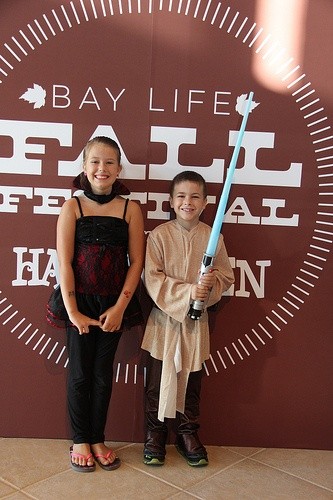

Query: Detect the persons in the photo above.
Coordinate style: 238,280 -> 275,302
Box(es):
140,171 -> 236,466
56,135 -> 145,473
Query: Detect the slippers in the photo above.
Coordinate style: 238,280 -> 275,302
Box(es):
69,444 -> 121,473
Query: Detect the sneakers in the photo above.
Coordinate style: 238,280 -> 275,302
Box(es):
176,442 -> 208,466
143,449 -> 165,465
